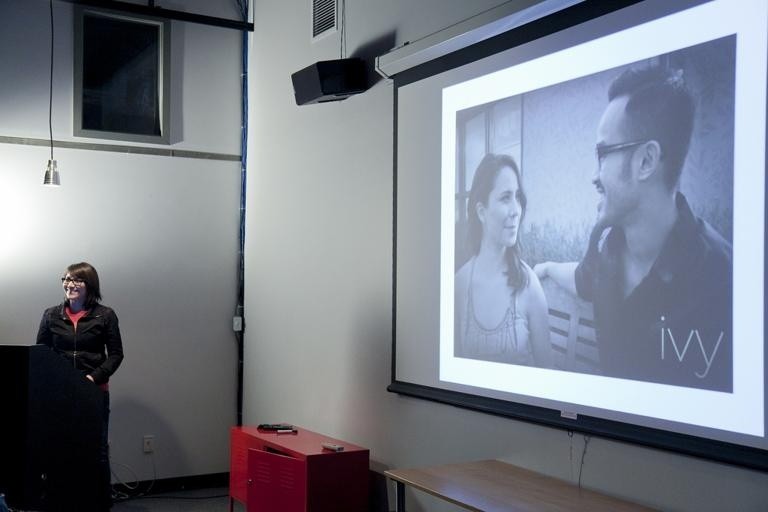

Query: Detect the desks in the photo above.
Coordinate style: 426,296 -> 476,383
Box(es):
384,458 -> 668,511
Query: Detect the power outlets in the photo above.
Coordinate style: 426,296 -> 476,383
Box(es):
142,435 -> 156,453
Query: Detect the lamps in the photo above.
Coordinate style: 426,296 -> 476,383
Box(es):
44,0 -> 61,185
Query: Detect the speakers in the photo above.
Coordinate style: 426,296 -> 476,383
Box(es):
291,58 -> 365,106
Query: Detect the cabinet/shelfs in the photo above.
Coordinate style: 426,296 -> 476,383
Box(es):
228,424 -> 370,512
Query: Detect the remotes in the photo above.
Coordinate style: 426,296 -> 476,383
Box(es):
323,442 -> 344,451
258,424 -> 293,431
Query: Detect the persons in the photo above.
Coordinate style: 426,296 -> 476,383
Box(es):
36,262 -> 124,512
532,67 -> 732,393
454,153 -> 549,367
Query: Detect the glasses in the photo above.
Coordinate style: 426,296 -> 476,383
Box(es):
595,140 -> 641,159
62,278 -> 84,285
